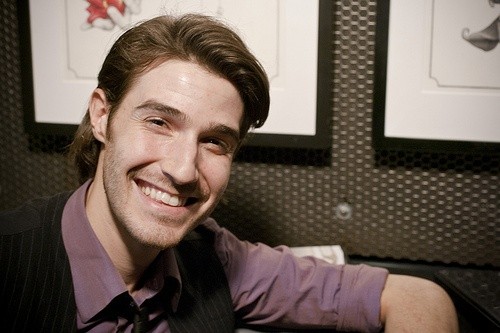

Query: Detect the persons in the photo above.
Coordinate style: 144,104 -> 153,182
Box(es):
0,13 -> 460,333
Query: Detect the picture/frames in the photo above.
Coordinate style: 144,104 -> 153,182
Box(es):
16,0 -> 334,148
372,0 -> 500,155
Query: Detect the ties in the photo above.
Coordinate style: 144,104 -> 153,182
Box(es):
116,293 -> 164,333
